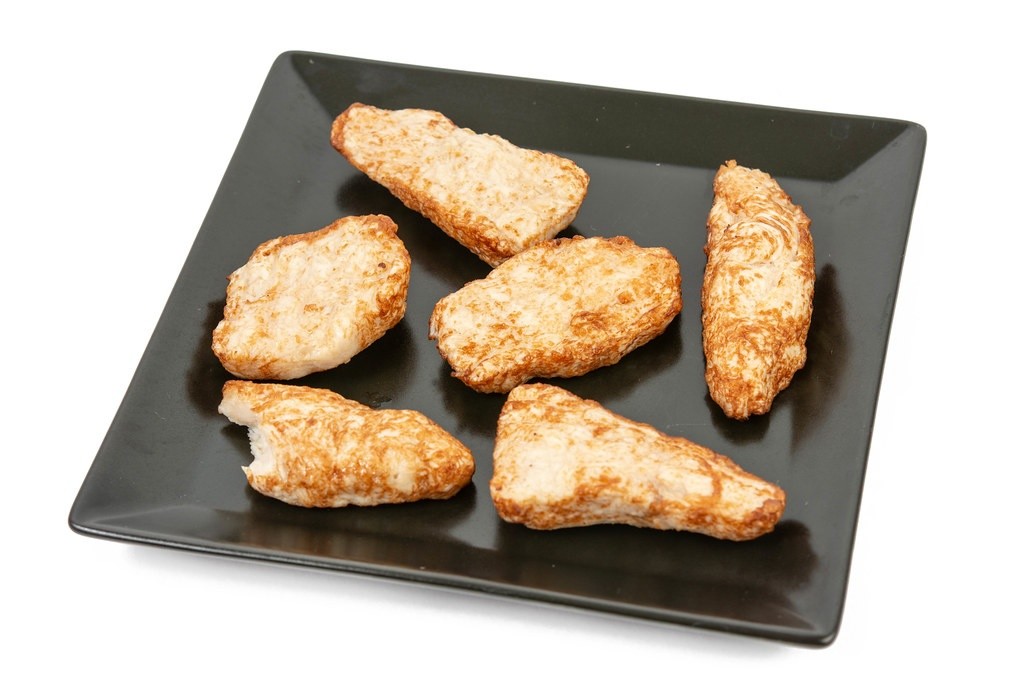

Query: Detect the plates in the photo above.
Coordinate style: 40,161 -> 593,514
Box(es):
65,49 -> 928,653
